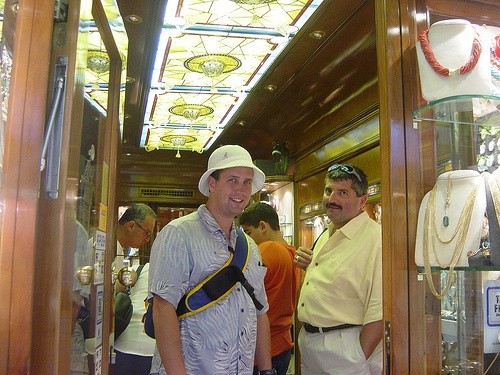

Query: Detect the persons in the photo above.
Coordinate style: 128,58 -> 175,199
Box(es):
72,219 -> 89,336
117,203 -> 157,269
113,263 -> 157,375
293,164 -> 384,375
239,202 -> 300,375
146,145 -> 273,375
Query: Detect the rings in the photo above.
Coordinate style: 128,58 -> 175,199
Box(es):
296,256 -> 299,259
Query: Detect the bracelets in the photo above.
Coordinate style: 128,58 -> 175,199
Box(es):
260,370 -> 274,375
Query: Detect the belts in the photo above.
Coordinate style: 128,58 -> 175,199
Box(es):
302,322 -> 357,334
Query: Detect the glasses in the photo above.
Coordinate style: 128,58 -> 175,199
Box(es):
328,164 -> 361,181
134,219 -> 153,239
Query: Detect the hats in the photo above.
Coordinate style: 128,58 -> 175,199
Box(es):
199,144 -> 266,198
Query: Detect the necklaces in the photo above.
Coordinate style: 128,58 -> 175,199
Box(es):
420,24 -> 481,76
488,174 -> 500,227
423,173 -> 480,298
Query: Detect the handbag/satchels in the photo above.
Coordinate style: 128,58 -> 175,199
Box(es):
115,291 -> 132,341
142,229 -> 250,339
